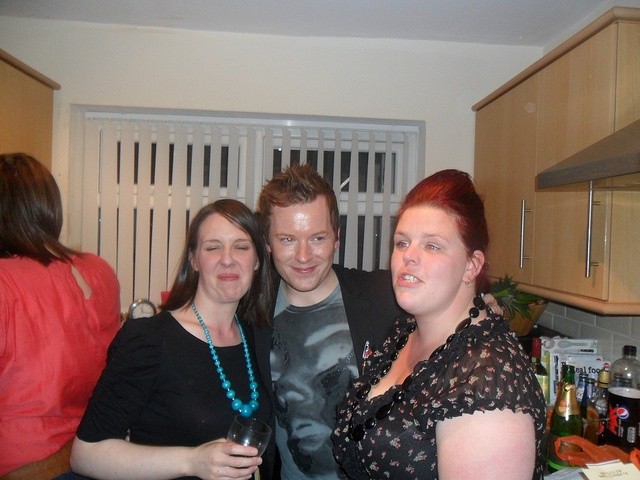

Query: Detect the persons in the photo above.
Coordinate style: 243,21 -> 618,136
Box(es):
1,152 -> 121,480
70,198 -> 275,480
330,169 -> 547,480
237,161 -> 504,480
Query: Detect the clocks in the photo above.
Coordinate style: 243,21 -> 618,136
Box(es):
127,298 -> 157,320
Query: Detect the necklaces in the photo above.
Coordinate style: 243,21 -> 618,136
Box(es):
190,300 -> 261,418
347,295 -> 486,441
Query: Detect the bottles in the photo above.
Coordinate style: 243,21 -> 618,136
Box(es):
607,344 -> 640,451
580,378 -> 597,420
576,372 -> 587,412
527,337 -> 550,405
584,370 -> 612,446
546,363 -> 582,474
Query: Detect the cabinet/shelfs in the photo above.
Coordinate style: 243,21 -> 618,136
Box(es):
471,6 -> 639,314
0,48 -> 62,176
